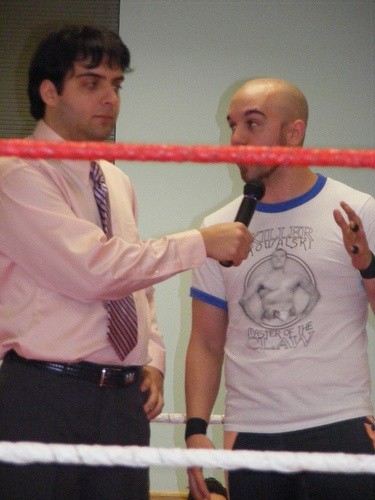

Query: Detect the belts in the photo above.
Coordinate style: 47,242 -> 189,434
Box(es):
3,348 -> 143,388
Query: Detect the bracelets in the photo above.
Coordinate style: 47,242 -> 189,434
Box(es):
185,416 -> 208,440
360,253 -> 375,279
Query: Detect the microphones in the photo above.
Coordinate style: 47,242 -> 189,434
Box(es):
218,179 -> 265,267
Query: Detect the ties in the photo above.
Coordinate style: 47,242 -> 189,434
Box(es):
87,160 -> 139,360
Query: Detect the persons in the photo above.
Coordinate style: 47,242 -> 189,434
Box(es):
0,22 -> 253,500
185,78 -> 375,500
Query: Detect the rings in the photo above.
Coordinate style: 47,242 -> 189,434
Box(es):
352,246 -> 358,254
350,224 -> 359,232
